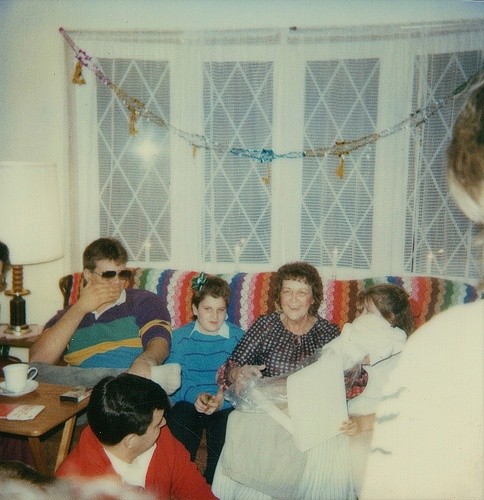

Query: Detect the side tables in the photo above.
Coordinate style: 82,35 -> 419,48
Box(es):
0,324 -> 45,363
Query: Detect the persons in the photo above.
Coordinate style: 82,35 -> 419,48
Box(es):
0,238 -> 424,500
364,81 -> 484,499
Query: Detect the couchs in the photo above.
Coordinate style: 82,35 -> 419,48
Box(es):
28,266 -> 484,476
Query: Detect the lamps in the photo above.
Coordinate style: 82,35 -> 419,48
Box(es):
0,160 -> 65,340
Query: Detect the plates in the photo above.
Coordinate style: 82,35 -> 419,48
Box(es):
0,380 -> 39,396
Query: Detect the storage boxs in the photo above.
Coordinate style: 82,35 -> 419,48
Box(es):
59,386 -> 92,403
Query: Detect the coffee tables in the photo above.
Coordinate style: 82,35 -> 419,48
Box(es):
0,377 -> 91,475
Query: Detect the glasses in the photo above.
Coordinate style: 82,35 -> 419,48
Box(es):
90,268 -> 132,281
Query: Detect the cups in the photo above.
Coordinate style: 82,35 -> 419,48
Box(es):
2,364 -> 38,392
